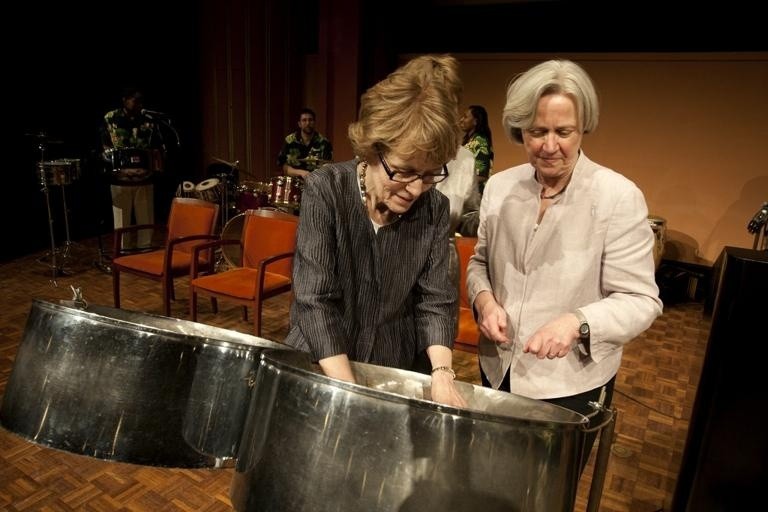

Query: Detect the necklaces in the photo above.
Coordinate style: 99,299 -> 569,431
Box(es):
360,161 -> 368,209
541,184 -> 567,200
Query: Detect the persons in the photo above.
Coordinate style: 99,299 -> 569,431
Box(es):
460,104 -> 494,197
432,145 -> 482,342
278,109 -> 335,183
101,85 -> 167,256
285,55 -> 473,411
463,60 -> 666,479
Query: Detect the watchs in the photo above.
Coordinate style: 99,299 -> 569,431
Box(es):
574,309 -> 589,339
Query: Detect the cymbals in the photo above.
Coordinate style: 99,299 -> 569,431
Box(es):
294,156 -> 333,164
213,157 -> 257,177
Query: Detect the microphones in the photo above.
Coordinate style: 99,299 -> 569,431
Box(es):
141,108 -> 166,119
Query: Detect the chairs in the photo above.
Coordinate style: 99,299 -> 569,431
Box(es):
110,197 -> 219,317
453,237 -> 495,354
191,209 -> 300,336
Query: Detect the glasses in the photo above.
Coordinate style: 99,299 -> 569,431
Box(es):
379,153 -> 449,184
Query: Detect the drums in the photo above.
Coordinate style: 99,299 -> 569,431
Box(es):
177,182 -> 196,197
234,182 -> 272,213
266,176 -> 303,209
647,215 -> 667,273
103,148 -> 165,187
196,178 -> 221,203
39,161 -> 72,186
58,158 -> 81,183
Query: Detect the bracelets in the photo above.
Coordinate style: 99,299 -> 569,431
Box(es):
432,365 -> 456,379
